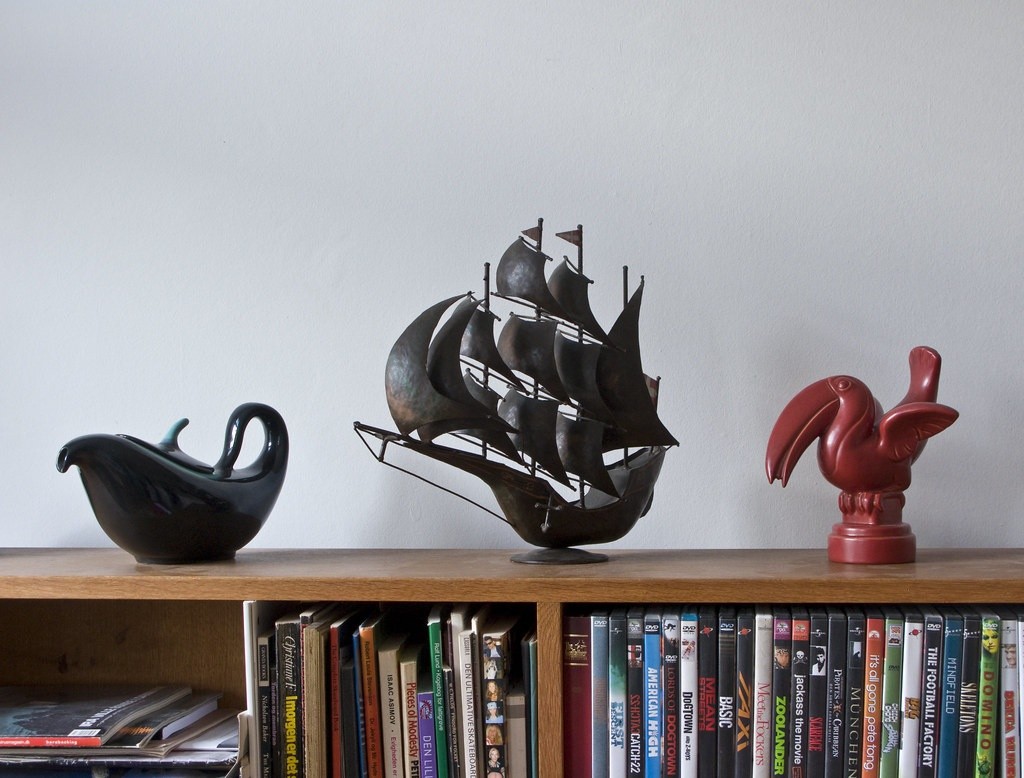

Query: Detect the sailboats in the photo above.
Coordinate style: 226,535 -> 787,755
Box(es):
354,216 -> 680,550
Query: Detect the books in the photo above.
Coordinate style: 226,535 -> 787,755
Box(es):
0,601 -> 1024,778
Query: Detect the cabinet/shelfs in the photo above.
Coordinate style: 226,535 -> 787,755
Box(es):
0,544 -> 1024,778
